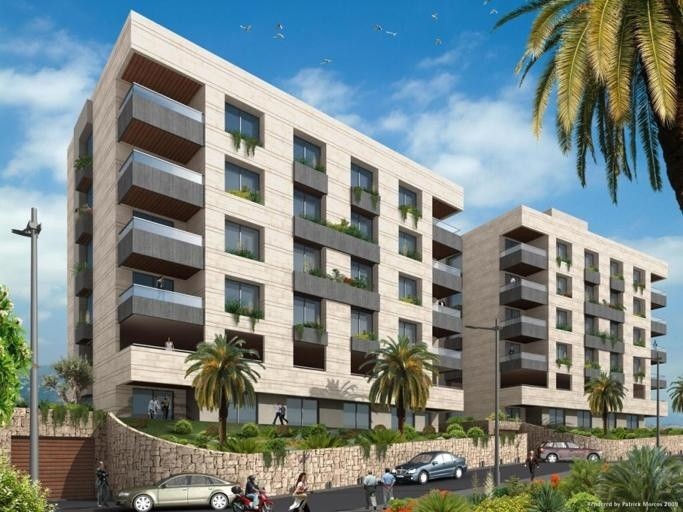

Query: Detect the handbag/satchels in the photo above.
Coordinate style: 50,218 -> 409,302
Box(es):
292,487 -> 307,499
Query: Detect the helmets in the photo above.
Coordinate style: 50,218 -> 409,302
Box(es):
249,474 -> 256,480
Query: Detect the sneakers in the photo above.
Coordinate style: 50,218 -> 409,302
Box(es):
383,507 -> 386,510
374,506 -> 376,510
